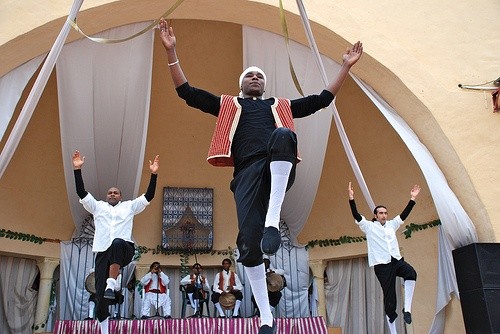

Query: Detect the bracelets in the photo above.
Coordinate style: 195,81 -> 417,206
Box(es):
167,60 -> 179,66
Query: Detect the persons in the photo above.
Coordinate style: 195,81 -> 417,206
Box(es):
160,15 -> 363,334
348,181 -> 422,334
140,262 -> 172,319
180,263 -> 209,318
73,150 -> 159,334
84,266 -> 135,320
250,258 -> 287,318
211,258 -> 245,318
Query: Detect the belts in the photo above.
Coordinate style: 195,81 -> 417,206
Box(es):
148,289 -> 160,293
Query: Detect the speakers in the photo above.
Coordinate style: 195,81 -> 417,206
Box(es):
452,243 -> 500,334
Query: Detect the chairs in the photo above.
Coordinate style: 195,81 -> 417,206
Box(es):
211,283 -> 246,318
142,274 -> 170,319
180,284 -> 211,318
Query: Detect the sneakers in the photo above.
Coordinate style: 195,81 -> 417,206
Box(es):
402,308 -> 412,324
259,318 -> 277,334
260,226 -> 280,256
104,289 -> 115,299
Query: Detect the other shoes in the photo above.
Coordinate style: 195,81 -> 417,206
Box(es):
218,315 -> 225,318
85,317 -> 93,320
232,316 -> 238,318
164,315 -> 171,318
113,316 -> 117,320
142,316 -> 147,319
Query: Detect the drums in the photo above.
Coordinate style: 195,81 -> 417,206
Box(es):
220,293 -> 235,310
85,272 -> 96,294
266,273 -> 286,292
